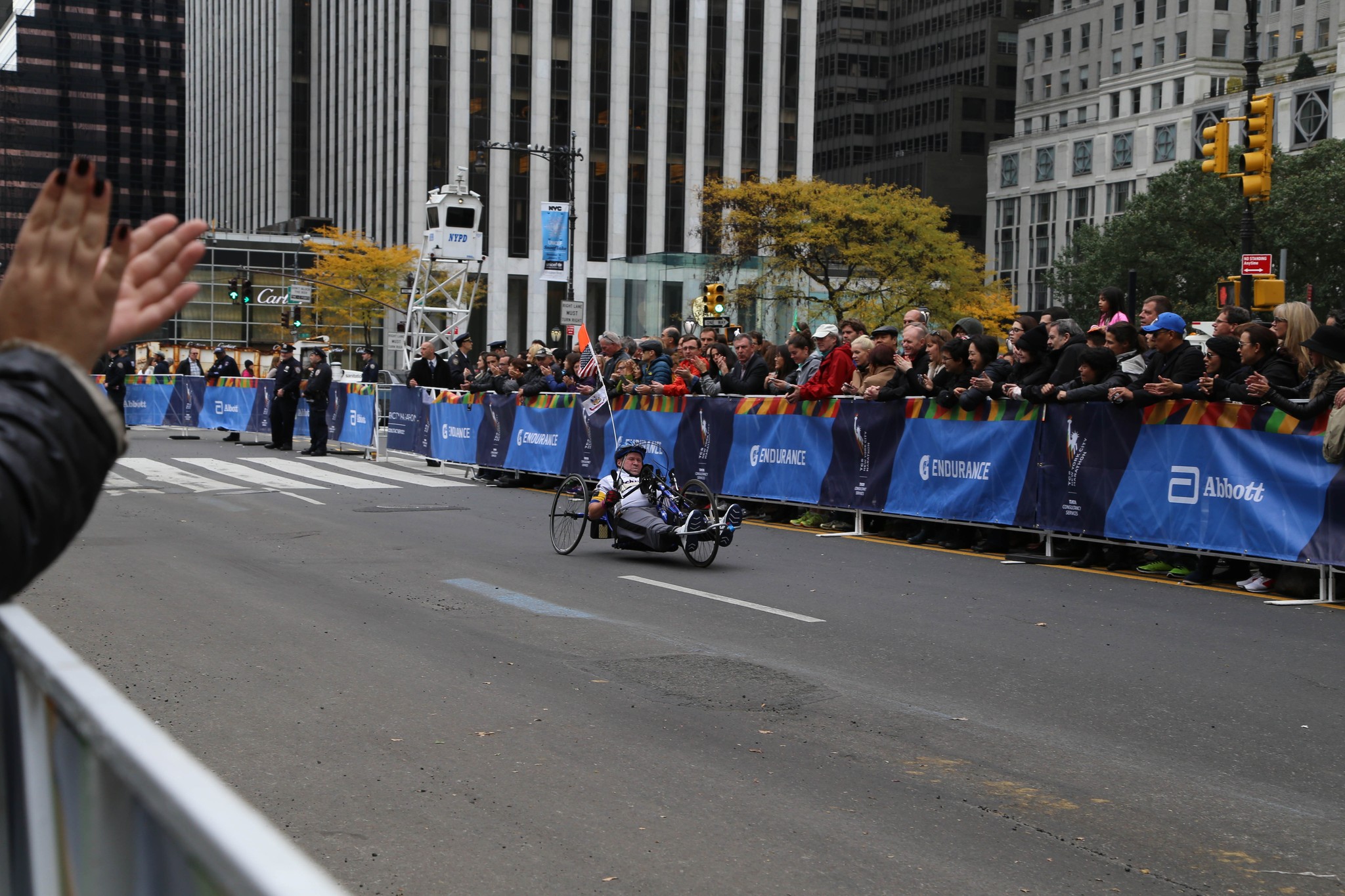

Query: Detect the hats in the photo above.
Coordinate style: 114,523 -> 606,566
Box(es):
1206,336 -> 1237,362
452,331 -> 553,358
1298,325 -> 1345,362
871,325 -> 899,337
103,344 -> 375,367
639,340 -> 663,354
1141,312 -> 1187,334
810,323 -> 840,338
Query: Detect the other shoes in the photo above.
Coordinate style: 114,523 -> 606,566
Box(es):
217,426 -> 229,431
223,432 -> 240,442
427,462 -> 440,468
311,448 -> 327,456
301,446 -> 317,455
264,441 -> 283,449
280,444 -> 292,451
470,468 -> 591,498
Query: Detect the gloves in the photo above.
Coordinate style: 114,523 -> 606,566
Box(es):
602,490 -> 621,510
674,485 -> 686,498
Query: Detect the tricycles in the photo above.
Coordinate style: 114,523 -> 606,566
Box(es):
549,441 -> 741,568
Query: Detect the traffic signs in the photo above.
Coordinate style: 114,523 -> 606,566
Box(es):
290,330 -> 297,334
703,317 -> 730,328
1241,254 -> 1271,275
400,287 -> 421,295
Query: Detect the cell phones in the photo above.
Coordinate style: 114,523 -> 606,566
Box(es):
844,383 -> 854,389
769,378 -> 778,383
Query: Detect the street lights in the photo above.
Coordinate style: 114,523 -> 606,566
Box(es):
294,235 -> 311,342
474,130 -> 584,383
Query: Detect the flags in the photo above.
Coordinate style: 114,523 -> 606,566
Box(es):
577,346 -> 596,380
581,385 -> 608,416
578,323 -> 590,352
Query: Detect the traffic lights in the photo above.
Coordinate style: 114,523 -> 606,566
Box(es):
1215,280 -> 1239,309
1239,98 -> 1273,196
228,279 -> 238,299
281,312 -> 288,329
1202,123 -> 1229,173
724,326 -> 742,343
703,283 -> 726,314
396,322 -> 405,333
241,279 -> 254,304
293,306 -> 301,327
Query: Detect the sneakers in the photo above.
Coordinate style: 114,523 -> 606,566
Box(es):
717,504 -> 743,547
680,509 -> 702,553
747,506 -> 1277,593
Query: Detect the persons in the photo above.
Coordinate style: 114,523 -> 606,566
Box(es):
0,155 -> 208,607
94,288 -> 1345,604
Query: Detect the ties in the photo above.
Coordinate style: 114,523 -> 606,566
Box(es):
429,361 -> 434,373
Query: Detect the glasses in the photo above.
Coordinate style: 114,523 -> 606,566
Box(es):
1204,352 -> 1220,359
616,366 -> 626,370
953,330 -> 966,335
498,363 -> 508,366
776,355 -> 783,358
1274,316 -> 1287,323
930,330 -> 947,342
642,349 -> 651,353
1010,327 -> 1025,332
814,335 -> 835,341
1215,319 -> 1229,323
682,347 -> 698,350
602,333 -> 616,343
1154,330 -> 1169,336
941,356 -> 953,360
1238,342 -> 1254,349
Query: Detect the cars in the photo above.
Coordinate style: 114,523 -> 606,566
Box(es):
374,370 -> 411,425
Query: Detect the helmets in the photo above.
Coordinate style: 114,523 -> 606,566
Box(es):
614,441 -> 647,468
